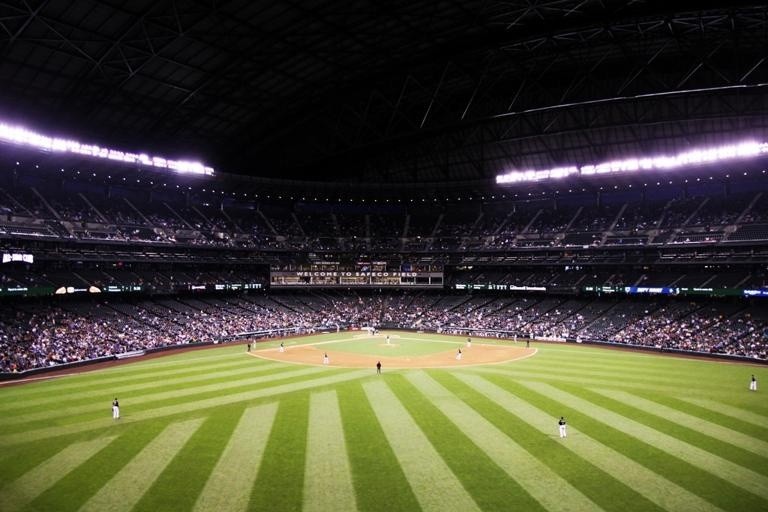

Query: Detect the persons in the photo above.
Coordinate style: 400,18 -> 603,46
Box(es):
748,374 -> 757,391
1,183 -> 768,376
558,416 -> 567,438
112,396 -> 120,419
